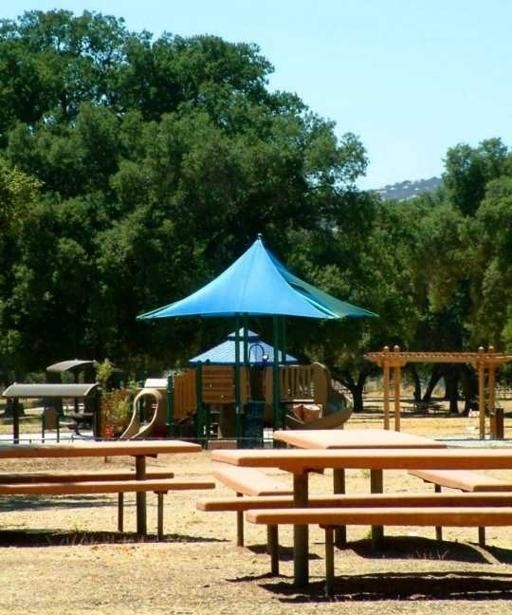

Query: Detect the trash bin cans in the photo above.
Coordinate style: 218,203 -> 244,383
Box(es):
237,401 -> 265,449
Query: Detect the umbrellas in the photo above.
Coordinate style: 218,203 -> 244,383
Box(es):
133,232 -> 382,437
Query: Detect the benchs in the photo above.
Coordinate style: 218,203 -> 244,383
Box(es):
0,471 -> 177,536
208,468 -> 295,546
2,479 -> 215,541
406,467 -> 511,547
244,507 -> 512,597
197,493 -> 511,545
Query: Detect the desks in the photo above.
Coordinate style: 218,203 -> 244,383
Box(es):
1,439 -> 200,540
210,449 -> 511,593
274,426 -> 448,546
